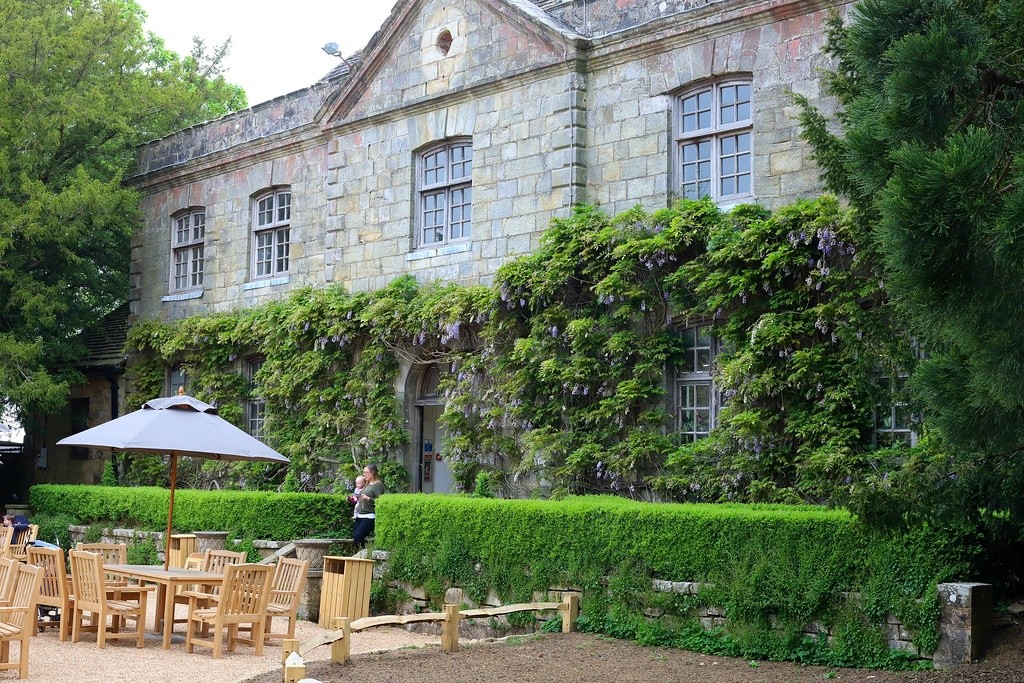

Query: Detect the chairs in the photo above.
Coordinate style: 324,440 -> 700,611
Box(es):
0,525 -> 309,680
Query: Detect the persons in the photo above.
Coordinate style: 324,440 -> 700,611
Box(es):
347,463 -> 384,554
3,514 -> 32,545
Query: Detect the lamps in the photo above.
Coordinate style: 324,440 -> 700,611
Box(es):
322,42 -> 351,68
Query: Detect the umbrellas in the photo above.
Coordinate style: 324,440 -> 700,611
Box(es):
56,387 -> 291,571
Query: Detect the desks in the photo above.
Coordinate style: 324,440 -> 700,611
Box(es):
103,564 -> 225,650
